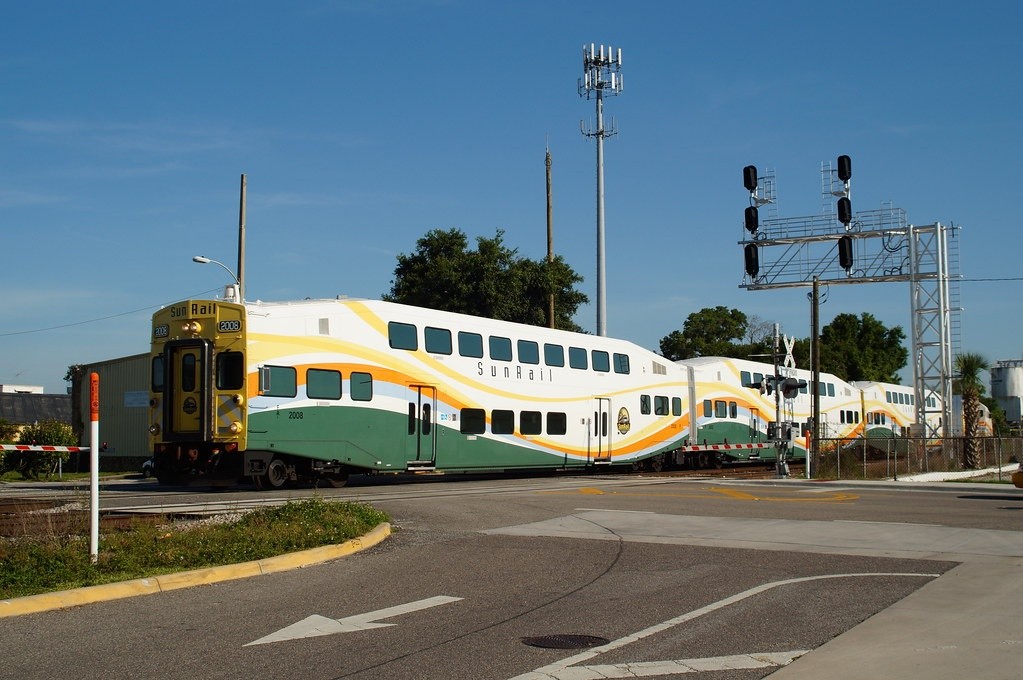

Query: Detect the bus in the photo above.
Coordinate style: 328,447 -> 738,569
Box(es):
148,298 -> 993,492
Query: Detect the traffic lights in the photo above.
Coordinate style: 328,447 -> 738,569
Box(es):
744,165 -> 759,280
837,155 -> 854,270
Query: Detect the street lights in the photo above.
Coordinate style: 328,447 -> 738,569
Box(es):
193,173 -> 247,304
